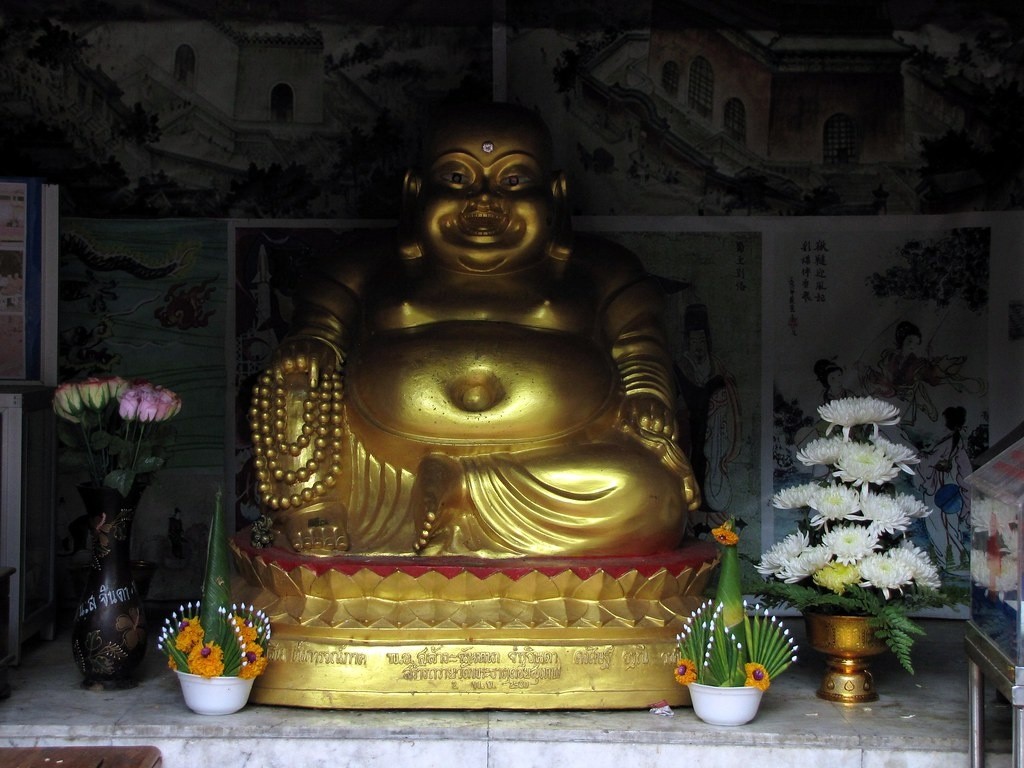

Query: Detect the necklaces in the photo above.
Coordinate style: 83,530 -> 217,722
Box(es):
248,350 -> 343,509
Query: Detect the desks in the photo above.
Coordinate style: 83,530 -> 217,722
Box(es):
960,619 -> 1024,768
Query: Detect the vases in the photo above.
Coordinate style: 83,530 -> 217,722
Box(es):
687,684 -> 764,730
74,486 -> 148,695
805,609 -> 883,706
173,668 -> 256,713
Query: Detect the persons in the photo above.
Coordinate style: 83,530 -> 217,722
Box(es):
232,101 -> 703,553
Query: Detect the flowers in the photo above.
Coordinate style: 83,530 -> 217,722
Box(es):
47,376 -> 189,495
159,497 -> 270,681
761,400 -> 949,678
673,523 -> 798,692
